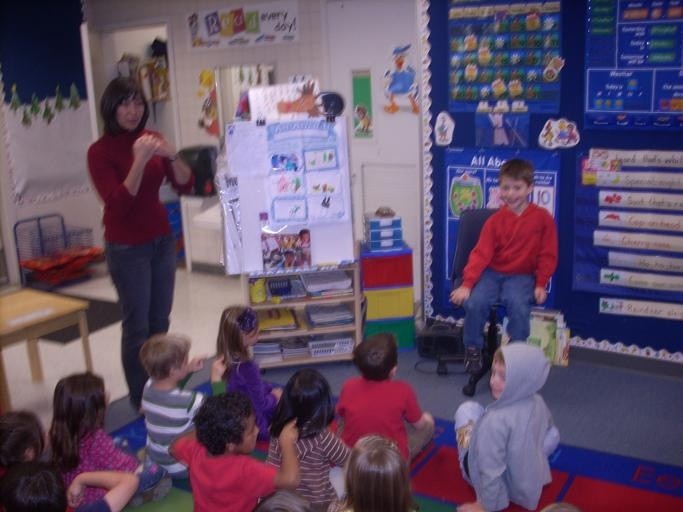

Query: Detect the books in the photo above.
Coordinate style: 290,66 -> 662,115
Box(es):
254,269 -> 355,365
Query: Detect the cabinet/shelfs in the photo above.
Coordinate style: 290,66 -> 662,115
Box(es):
361,211 -> 405,251
242,260 -> 367,366
360,246 -> 415,351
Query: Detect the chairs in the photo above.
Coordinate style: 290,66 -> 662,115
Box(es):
436,210 -> 505,399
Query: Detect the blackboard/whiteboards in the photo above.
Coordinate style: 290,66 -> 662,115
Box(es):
224,116 -> 359,278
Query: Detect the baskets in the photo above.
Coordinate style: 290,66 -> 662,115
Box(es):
309,338 -> 353,357
269,279 -> 292,295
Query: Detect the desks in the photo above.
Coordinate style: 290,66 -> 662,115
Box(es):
0,287 -> 92,413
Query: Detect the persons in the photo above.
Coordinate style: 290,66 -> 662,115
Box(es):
454,341 -> 560,512
87,77 -> 195,415
448,160 -> 560,373
0,303 -> 435,512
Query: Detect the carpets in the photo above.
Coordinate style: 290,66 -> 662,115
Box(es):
23,340 -> 682,511
38,294 -> 122,345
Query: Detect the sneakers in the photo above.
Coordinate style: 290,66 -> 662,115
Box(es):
464,347 -> 483,374
128,478 -> 172,509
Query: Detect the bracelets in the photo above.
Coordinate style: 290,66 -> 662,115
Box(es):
168,153 -> 180,162
